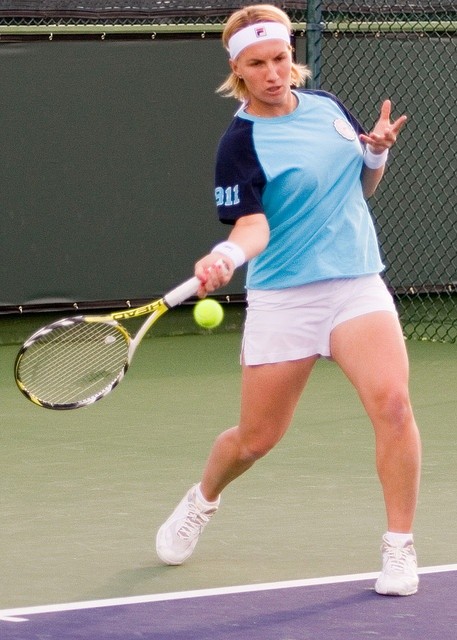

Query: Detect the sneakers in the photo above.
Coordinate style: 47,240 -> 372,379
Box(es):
154,485 -> 219,566
375,537 -> 420,598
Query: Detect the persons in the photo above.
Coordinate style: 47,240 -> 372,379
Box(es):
154,5 -> 419,595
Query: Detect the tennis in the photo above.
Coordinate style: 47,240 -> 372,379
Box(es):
192,298 -> 223,330
